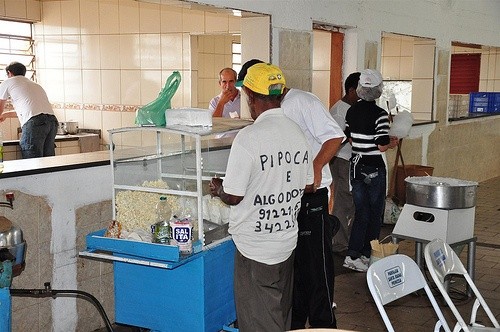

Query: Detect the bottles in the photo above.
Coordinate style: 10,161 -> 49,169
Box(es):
153,196 -> 172,245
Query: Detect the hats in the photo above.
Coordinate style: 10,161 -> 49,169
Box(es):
234,63 -> 285,96
360,70 -> 383,89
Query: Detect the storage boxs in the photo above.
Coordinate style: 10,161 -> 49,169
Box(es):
469,92 -> 500,114
448,94 -> 470,118
165,107 -> 212,127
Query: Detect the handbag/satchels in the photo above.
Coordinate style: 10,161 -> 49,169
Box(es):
370,235 -> 397,267
133,71 -> 182,127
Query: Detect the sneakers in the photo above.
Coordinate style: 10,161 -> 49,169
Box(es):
343,256 -> 370,271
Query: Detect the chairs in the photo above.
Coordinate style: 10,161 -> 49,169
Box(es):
367,254 -> 452,332
425,238 -> 500,332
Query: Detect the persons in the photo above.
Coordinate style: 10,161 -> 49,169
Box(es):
329,72 -> 361,257
210,67 -> 242,118
238,59 -> 345,329
208,64 -> 315,332
0,61 -> 59,159
342,68 -> 399,273
